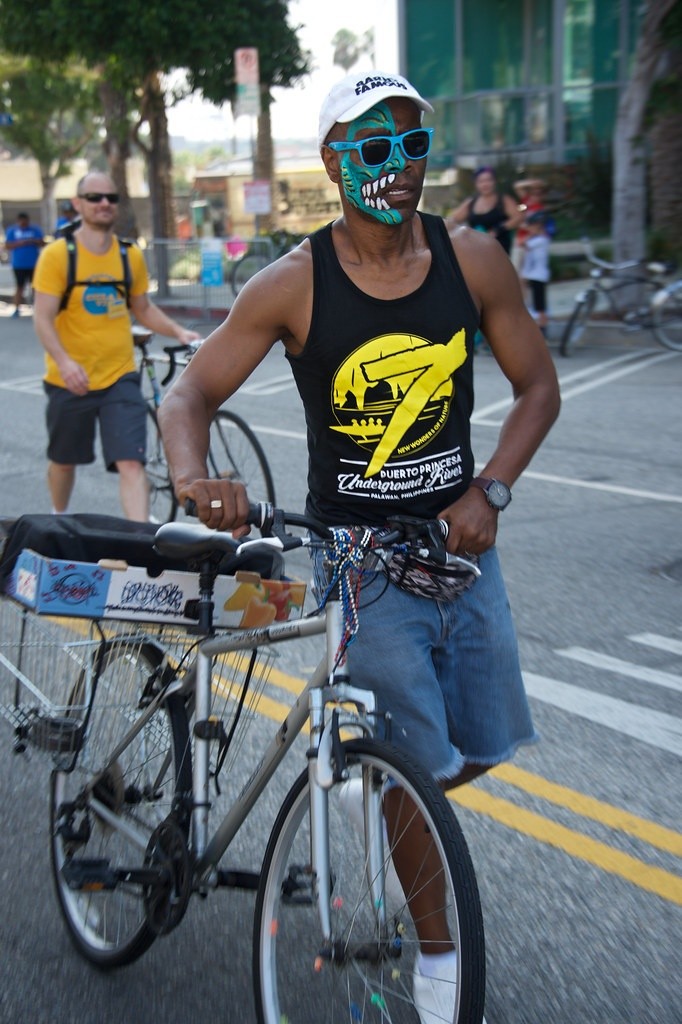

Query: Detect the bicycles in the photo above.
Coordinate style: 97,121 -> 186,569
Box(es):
559,237 -> 681,359
47,499 -> 493,1024
231,229 -> 304,297
126,325 -> 279,523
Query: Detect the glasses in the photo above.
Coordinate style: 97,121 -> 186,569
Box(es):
79,192 -> 121,205
327,128 -> 437,168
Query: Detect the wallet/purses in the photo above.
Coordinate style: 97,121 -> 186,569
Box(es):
374,528 -> 486,603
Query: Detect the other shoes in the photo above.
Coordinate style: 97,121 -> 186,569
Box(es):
411,958 -> 487,1024
340,777 -> 412,919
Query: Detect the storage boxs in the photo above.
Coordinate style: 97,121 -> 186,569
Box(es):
0,513 -> 309,630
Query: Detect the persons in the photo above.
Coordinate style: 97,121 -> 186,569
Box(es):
517,210 -> 551,341
31,172 -> 202,524
54,202 -> 82,239
157,72 -> 561,1024
511,178 -> 549,273
5,213 -> 45,318
446,166 -> 527,354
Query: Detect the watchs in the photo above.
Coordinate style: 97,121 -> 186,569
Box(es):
469,477 -> 512,511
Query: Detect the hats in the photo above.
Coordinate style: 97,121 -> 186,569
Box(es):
318,69 -> 434,157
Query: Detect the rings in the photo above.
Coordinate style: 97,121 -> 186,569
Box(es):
211,500 -> 221,508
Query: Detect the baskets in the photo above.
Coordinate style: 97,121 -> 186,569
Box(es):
0,595 -> 282,778
